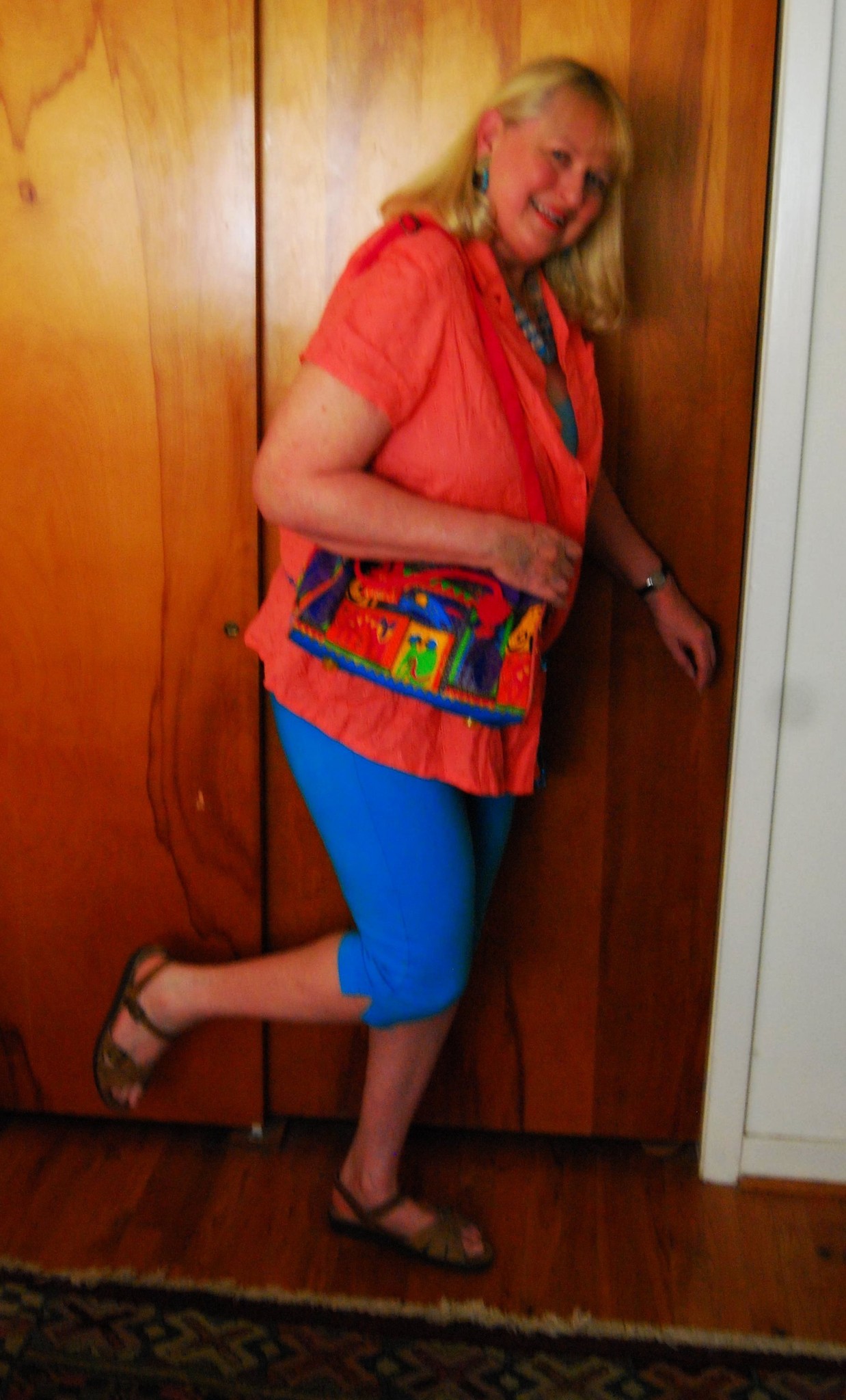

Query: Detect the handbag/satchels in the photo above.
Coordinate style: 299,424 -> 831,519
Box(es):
290,540 -> 549,725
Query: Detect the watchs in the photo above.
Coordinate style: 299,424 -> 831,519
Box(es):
634,560 -> 672,598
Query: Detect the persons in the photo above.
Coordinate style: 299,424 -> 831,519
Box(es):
87,51 -> 722,1273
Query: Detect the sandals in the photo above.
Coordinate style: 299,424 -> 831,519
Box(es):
92,944 -> 181,1108
330,1169 -> 495,1274
